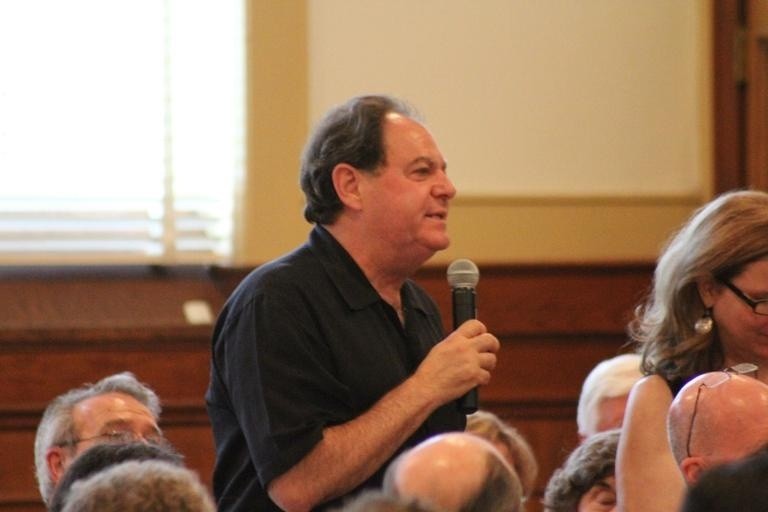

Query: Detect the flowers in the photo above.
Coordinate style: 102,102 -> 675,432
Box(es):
446,259 -> 480,412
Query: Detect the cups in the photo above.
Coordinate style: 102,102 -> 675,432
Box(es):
57,428 -> 173,454
714,273 -> 768,317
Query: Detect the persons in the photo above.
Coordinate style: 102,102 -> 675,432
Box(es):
550,422 -> 581,470
576,352 -> 656,445
49,443 -> 186,511
614,190 -> 768,511
545,428 -> 622,511
465,411 -> 537,503
61,458 -> 217,511
344,488 -> 441,511
201,94 -> 499,511
33,370 -> 162,511
668,362 -> 766,486
384,433 -> 522,512
682,443 -> 766,512
